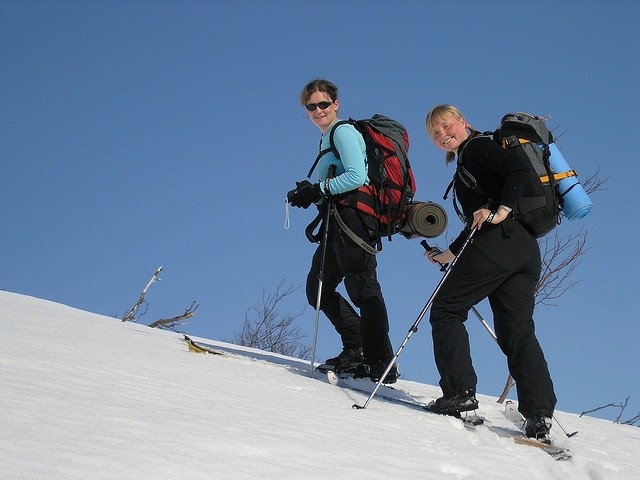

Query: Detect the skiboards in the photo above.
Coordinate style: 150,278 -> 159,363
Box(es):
183,332 -> 230,359
325,370 -> 576,462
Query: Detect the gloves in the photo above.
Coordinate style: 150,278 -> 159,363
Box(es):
285,180 -> 326,209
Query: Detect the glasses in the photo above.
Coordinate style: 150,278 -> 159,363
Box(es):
305,100 -> 334,111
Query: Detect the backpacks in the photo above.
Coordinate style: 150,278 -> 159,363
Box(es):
307,113 -> 416,243
443,112 -> 564,237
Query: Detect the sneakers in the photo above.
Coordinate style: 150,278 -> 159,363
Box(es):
435,388 -> 479,414
336,360 -> 397,384
525,414 -> 552,438
326,346 -> 364,366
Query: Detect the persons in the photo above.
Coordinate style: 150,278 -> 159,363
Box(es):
420,104 -> 558,444
284,79 -> 397,384
